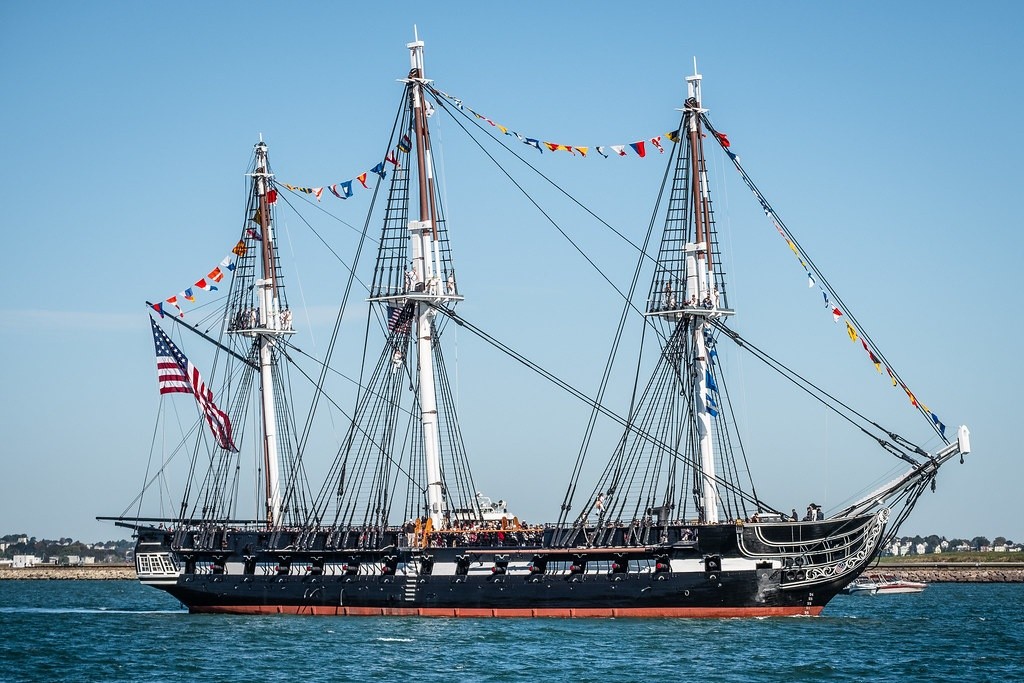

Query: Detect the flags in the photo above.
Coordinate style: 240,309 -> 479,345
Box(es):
704,130 -> 946,436
145,185 -> 279,318
278,119 -> 417,203
439,90 -> 681,159
148,313 -> 240,455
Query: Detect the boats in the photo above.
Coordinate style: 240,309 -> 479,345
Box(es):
842,570 -> 929,595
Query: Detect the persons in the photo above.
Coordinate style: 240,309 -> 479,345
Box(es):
148,492 -> 825,550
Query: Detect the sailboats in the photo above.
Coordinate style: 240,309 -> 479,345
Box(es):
95,23 -> 970,617
